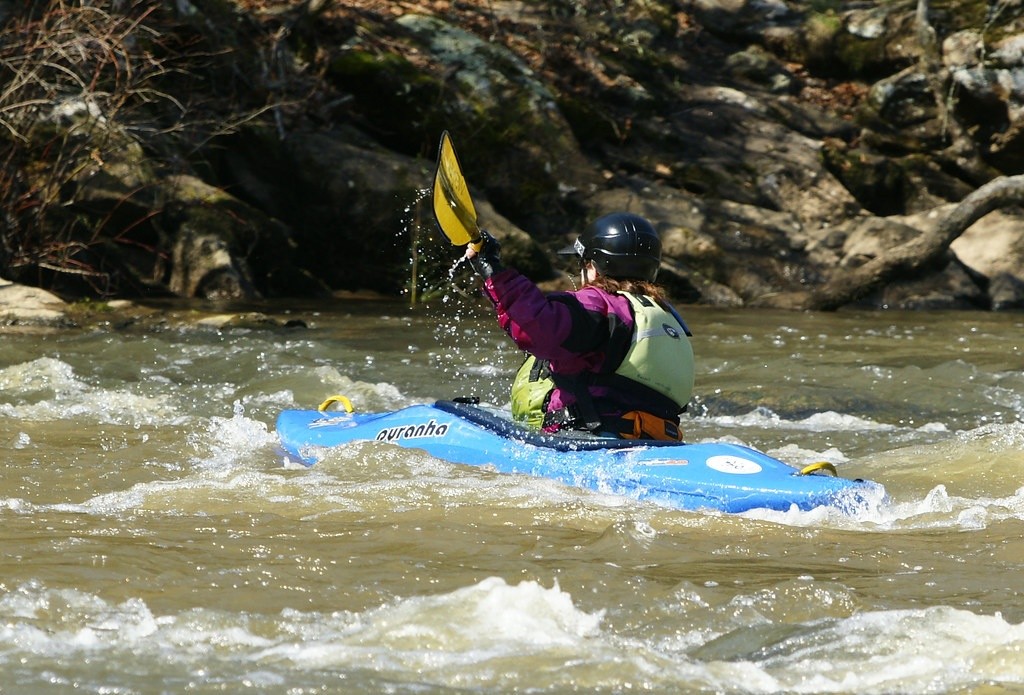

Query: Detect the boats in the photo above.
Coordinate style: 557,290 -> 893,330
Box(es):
274,394 -> 886,519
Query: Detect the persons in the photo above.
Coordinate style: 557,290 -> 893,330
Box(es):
466,212 -> 696,442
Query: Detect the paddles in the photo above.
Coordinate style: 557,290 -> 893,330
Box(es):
435,129 -> 481,267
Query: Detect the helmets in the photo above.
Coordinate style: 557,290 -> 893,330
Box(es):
558,211 -> 662,282
